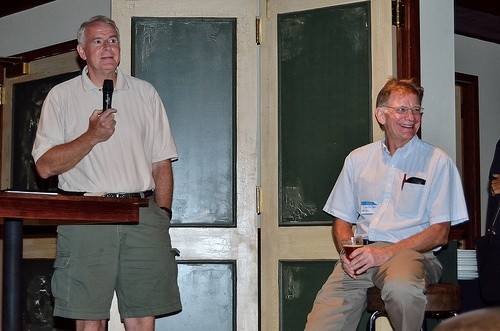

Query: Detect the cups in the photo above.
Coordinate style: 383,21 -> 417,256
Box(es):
340,236 -> 368,277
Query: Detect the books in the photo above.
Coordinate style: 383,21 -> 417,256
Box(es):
457,250 -> 478,280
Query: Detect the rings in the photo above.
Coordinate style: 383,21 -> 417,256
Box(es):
491,191 -> 494,194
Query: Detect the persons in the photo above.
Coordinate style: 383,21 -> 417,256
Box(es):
304,77 -> 469,331
31,15 -> 182,331
486,139 -> 500,234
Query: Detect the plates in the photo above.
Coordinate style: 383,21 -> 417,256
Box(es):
457,249 -> 478,281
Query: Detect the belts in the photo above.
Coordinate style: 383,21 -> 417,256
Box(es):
57,188 -> 154,199
356,239 -> 376,246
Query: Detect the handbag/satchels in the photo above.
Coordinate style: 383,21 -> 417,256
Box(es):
477,205 -> 500,306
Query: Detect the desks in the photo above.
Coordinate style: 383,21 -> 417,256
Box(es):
0,189 -> 150,331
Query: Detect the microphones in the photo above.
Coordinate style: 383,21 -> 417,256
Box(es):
102,79 -> 114,112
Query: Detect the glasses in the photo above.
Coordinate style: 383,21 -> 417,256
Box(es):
383,105 -> 424,114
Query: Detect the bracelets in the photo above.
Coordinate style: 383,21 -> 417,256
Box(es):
159,206 -> 173,221
340,250 -> 346,254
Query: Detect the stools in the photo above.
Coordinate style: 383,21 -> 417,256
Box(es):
364,284 -> 463,330
